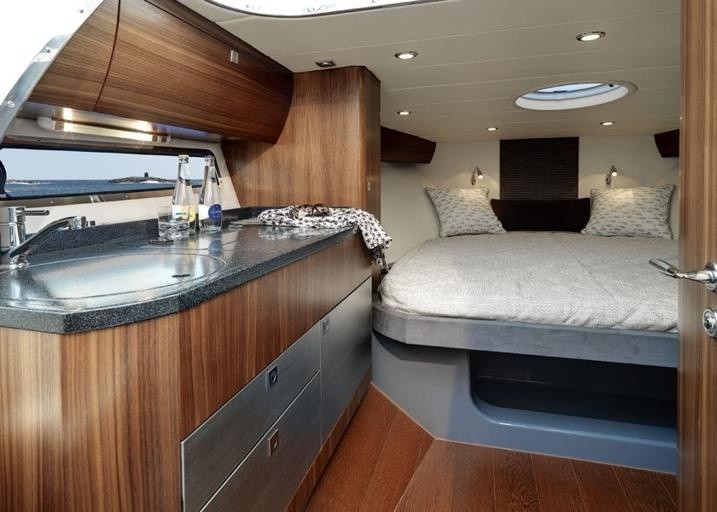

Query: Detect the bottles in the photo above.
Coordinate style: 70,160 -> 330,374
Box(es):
170,154 -> 196,234
197,155 -> 222,233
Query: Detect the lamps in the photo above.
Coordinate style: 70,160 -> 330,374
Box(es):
471,167 -> 483,185
51,107 -> 165,134
605,165 -> 618,185
37,117 -> 171,146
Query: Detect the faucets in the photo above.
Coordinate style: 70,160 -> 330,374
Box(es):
0,205 -> 96,271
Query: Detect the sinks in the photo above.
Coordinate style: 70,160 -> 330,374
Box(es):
0,252 -> 222,310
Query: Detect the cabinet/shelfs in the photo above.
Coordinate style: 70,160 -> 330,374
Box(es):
181,233 -> 373,512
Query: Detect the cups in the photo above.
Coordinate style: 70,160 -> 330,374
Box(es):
154,204 -> 189,239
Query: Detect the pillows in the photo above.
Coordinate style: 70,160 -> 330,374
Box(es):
490,197 -> 590,235
425,186 -> 508,236
581,184 -> 675,241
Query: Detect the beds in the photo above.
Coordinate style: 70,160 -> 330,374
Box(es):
372,230 -> 681,480
